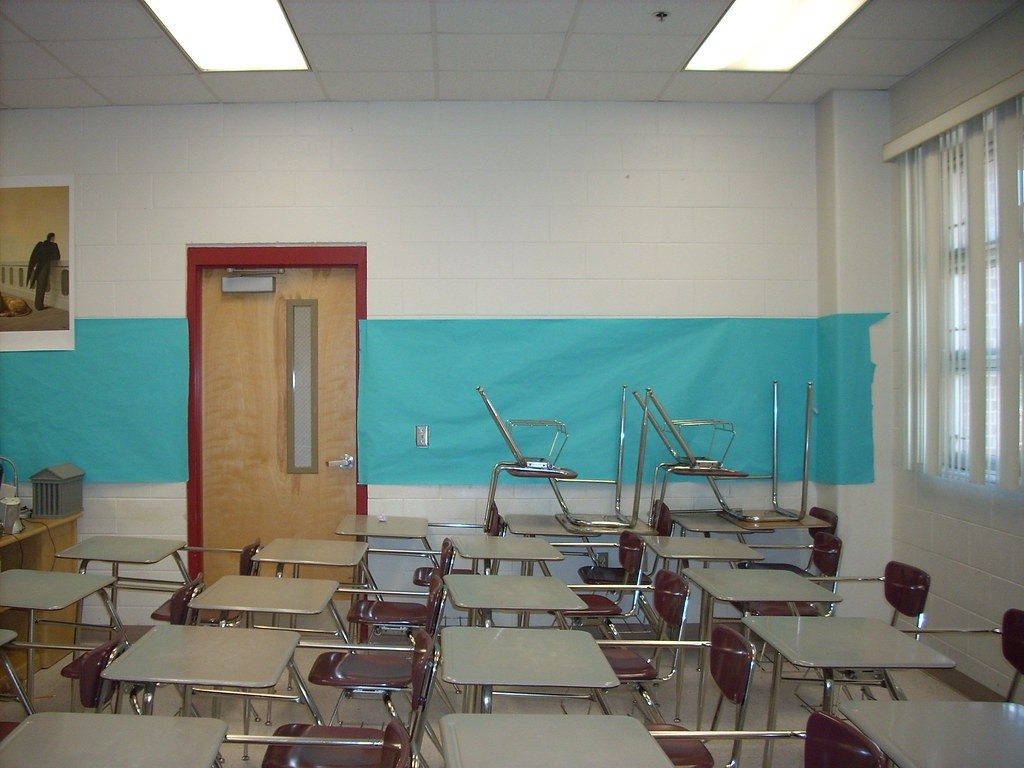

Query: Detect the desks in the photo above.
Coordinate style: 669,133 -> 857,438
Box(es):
0,569 -> 132,705
740,615 -> 957,768
441,574 -> 589,713
0,512 -> 85,692
837,700 -> 1024,768
101,625 -> 324,759
0,713 -> 227,768
640,536 -> 766,672
440,714 -> 675,768
55,536 -> 193,659
674,568 -> 844,731
187,575 -> 357,724
450,536 -> 565,627
502,513 -> 601,576
442,626 -> 620,715
250,537 -> 383,654
334,514 -> 439,641
671,513 -> 774,568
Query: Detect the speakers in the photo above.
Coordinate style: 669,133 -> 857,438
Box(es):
0,497 -> 25,535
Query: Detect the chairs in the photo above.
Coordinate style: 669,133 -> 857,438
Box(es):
60,381 -> 1024,768
